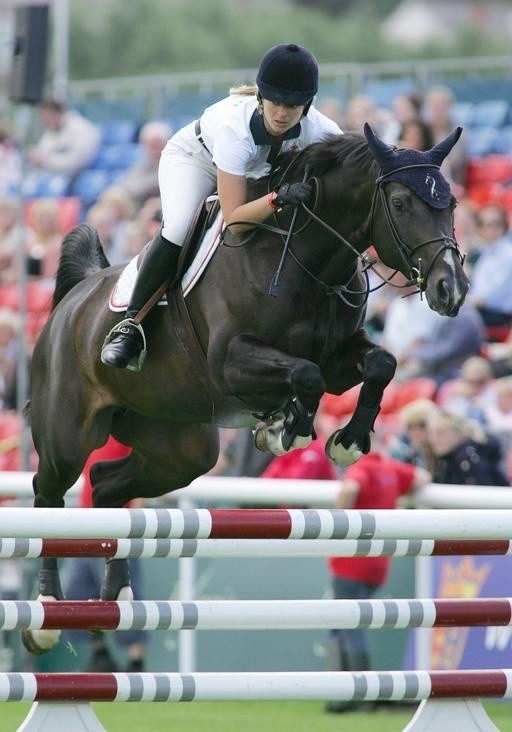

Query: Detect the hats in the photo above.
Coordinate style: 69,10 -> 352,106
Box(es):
256,44 -> 319,106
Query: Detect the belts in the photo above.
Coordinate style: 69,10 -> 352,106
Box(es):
194,120 -> 209,152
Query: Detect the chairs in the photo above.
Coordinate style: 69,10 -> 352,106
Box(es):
0,77 -> 510,334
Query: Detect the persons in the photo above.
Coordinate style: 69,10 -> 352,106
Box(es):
327,430 -> 429,711
1,86 -> 512,485
100,46 -> 346,368
61,433 -> 148,670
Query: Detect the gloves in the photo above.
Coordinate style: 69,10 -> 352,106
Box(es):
273,183 -> 312,206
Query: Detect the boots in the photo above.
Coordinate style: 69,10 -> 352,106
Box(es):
101,225 -> 182,369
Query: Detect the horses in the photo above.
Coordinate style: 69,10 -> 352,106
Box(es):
26,122 -> 473,656
